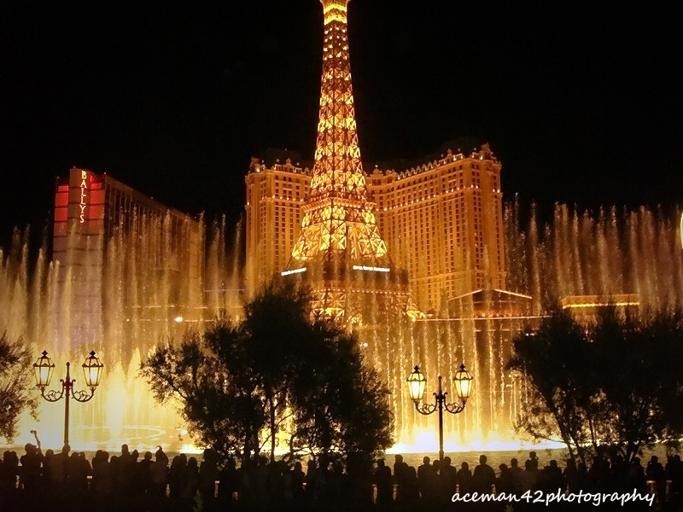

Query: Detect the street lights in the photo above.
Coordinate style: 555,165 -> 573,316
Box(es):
33,350 -> 104,455
406,363 -> 473,462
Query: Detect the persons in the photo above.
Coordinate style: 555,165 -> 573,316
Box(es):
0,441 -> 681,511
